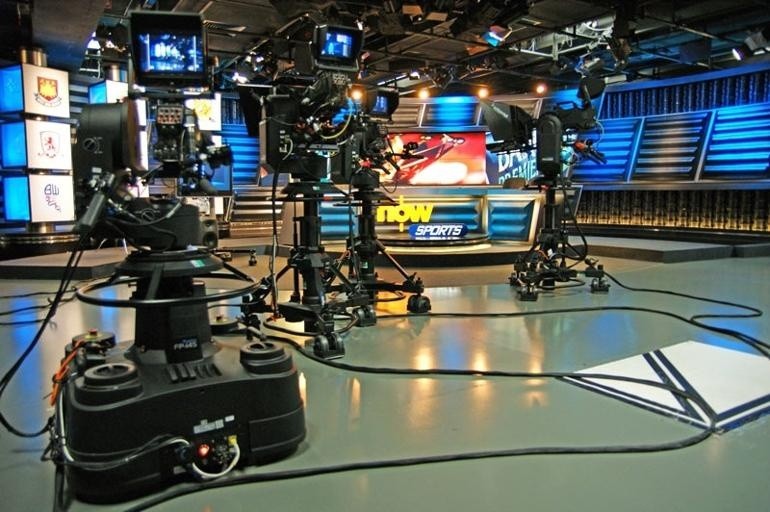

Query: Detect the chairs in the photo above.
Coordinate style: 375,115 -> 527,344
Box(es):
503,178 -> 526,188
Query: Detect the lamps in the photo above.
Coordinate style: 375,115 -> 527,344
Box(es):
732,46 -> 750,61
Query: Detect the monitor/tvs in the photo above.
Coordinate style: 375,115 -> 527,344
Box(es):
369,94 -> 389,115
318,27 -> 356,63
133,20 -> 205,80
